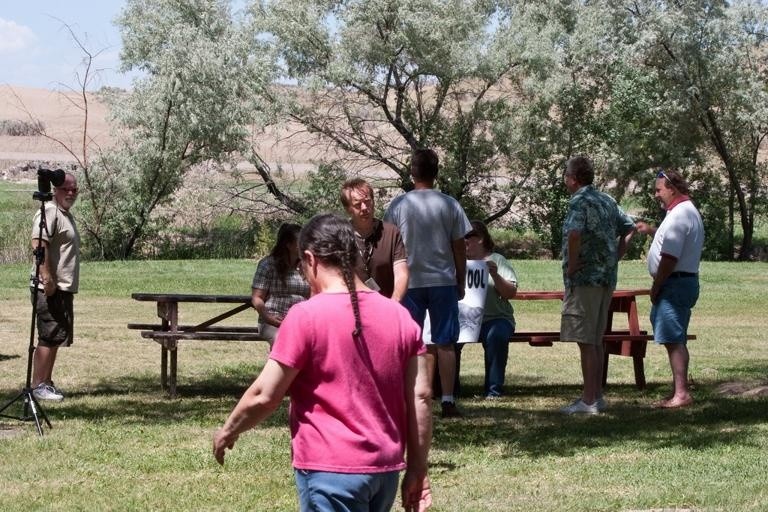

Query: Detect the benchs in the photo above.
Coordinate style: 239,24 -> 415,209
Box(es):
131,330 -> 695,396
128,324 -> 258,386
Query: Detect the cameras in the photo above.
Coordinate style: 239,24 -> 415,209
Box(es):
36,168 -> 66,192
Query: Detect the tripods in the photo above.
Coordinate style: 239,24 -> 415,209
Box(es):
0,193 -> 53,437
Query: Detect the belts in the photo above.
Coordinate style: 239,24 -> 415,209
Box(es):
669,271 -> 698,278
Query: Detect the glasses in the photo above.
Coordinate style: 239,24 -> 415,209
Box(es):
656,171 -> 673,184
464,232 -> 478,239
62,186 -> 80,193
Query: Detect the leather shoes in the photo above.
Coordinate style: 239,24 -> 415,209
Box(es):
442,399 -> 460,417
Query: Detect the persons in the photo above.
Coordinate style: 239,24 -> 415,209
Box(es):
213,214 -> 431,512
382,149 -> 473,417
431,220 -> 519,399
340,177 -> 409,302
637,169 -> 707,409
25,172 -> 80,402
559,156 -> 638,414
250,223 -> 317,349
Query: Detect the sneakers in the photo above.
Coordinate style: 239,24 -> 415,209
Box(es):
33,383 -> 64,403
560,400 -> 599,415
598,396 -> 608,411
